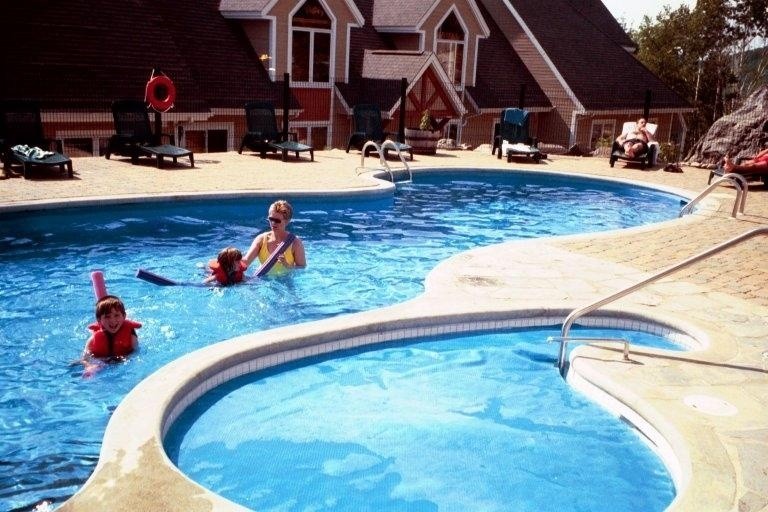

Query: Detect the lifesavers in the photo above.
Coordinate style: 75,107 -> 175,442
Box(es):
145,75 -> 177,112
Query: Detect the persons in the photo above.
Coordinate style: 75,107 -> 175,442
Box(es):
203,247 -> 247,286
615,118 -> 653,158
81,295 -> 142,367
724,149 -> 768,173
242,199 -> 306,269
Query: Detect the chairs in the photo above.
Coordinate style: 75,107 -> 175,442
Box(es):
492,107 -> 540,163
346,104 -> 413,161
0,100 -> 72,178
609,121 -> 658,170
106,101 -> 194,168
708,156 -> 768,189
239,102 -> 314,161
